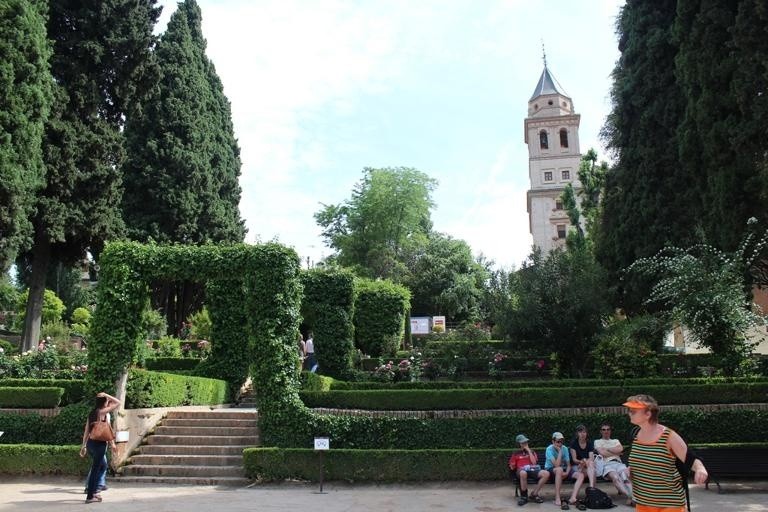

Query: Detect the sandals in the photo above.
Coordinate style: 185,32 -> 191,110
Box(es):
576,500 -> 586,510
561,498 -> 569,510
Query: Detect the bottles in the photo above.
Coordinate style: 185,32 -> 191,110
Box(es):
521,465 -> 541,473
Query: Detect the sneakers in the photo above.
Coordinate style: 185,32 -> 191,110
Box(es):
529,493 -> 543,502
518,497 -> 528,506
86,496 -> 102,502
84,484 -> 107,493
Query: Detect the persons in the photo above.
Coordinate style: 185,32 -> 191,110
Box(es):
78,389 -> 121,504
544,430 -> 586,506
298,331 -> 316,372
593,425 -> 633,502
623,394 -> 708,512
510,434 -> 551,506
569,423 -> 597,486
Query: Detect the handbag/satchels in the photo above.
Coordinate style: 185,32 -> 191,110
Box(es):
582,487 -> 616,509
90,421 -> 116,441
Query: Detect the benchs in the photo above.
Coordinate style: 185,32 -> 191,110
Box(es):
692,449 -> 768,490
508,453 -> 626,497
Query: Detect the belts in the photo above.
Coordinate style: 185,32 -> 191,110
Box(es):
610,459 -> 621,462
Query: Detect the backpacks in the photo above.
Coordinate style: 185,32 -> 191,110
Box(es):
637,430 -> 697,489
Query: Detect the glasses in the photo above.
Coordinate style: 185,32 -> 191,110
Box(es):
601,429 -> 610,431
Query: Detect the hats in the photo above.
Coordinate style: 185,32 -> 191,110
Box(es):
552,432 -> 564,440
622,401 -> 657,409
516,435 -> 530,443
